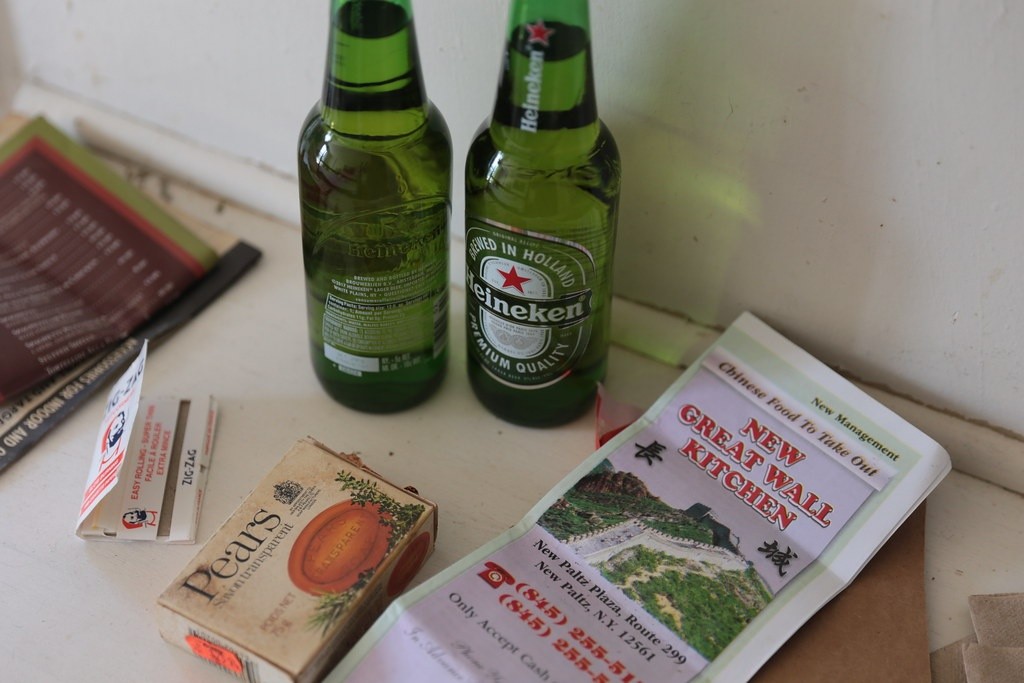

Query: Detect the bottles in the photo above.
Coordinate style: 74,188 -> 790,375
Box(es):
298,0 -> 453,414
464,0 -> 623,428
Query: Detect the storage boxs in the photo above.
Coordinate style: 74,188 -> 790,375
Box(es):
153,436 -> 439,683
